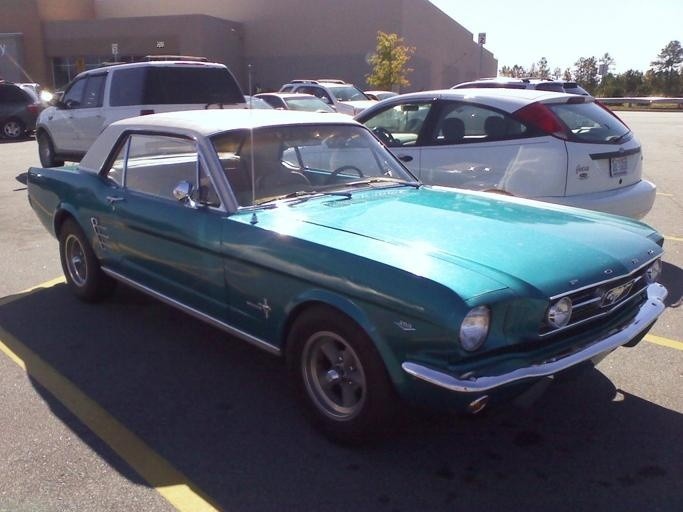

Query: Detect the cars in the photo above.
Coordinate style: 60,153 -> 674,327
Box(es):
242,78 -> 430,129
26,107 -> 667,447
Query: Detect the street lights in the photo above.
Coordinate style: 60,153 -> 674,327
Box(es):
248,64 -> 252,95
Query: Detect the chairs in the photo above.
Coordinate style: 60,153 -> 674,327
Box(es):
481,114 -> 507,141
436,116 -> 464,145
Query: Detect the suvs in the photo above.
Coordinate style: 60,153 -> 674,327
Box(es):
396,77 -> 596,135
281,86 -> 656,221
36,53 -> 249,169
0,82 -> 65,141
